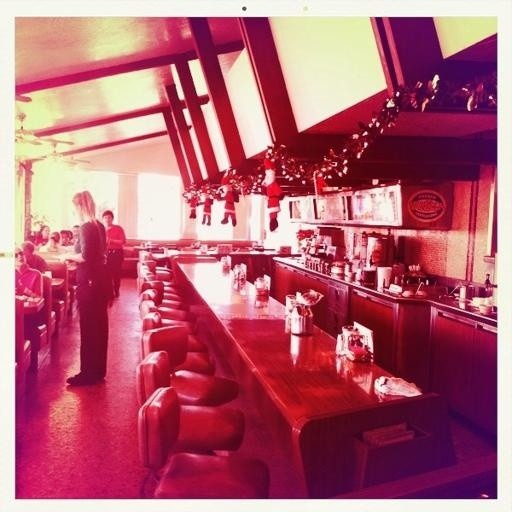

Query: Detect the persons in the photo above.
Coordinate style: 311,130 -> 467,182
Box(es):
35,226 -> 49,247
66,191 -> 109,386
217,174 -> 237,226
15,248 -> 43,369
262,158 -> 284,231
60,231 -> 73,246
201,187 -> 212,225
39,232 -> 66,255
102,211 -> 126,299
71,225 -> 81,242
21,241 -> 53,278
188,186 -> 197,219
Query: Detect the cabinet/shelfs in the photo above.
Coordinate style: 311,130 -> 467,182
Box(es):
273,256 -> 350,340
428,300 -> 497,443
350,284 -> 429,380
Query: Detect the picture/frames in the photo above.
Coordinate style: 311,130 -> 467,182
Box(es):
284,180 -> 403,226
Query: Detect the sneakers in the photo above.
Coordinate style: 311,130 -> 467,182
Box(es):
66,373 -> 95,386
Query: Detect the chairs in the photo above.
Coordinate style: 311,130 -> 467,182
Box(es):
137,262 -> 169,271
139,289 -> 198,321
140,300 -> 208,351
145,272 -> 178,294
136,350 -> 246,455
143,269 -> 174,287
47,263 -> 67,337
140,325 -> 240,406
140,265 -> 170,280
138,386 -> 270,499
142,312 -> 216,375
30,276 -> 52,373
144,276 -> 180,301
140,281 -> 183,309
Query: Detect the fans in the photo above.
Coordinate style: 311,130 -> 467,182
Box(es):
15,111 -> 43,145
41,142 -> 80,165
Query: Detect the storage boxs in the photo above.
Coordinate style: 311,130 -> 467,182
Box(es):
350,420 -> 431,488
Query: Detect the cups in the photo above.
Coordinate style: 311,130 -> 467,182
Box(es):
376,266 -> 392,293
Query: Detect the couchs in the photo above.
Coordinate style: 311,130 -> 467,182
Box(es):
120,247 -> 139,275
